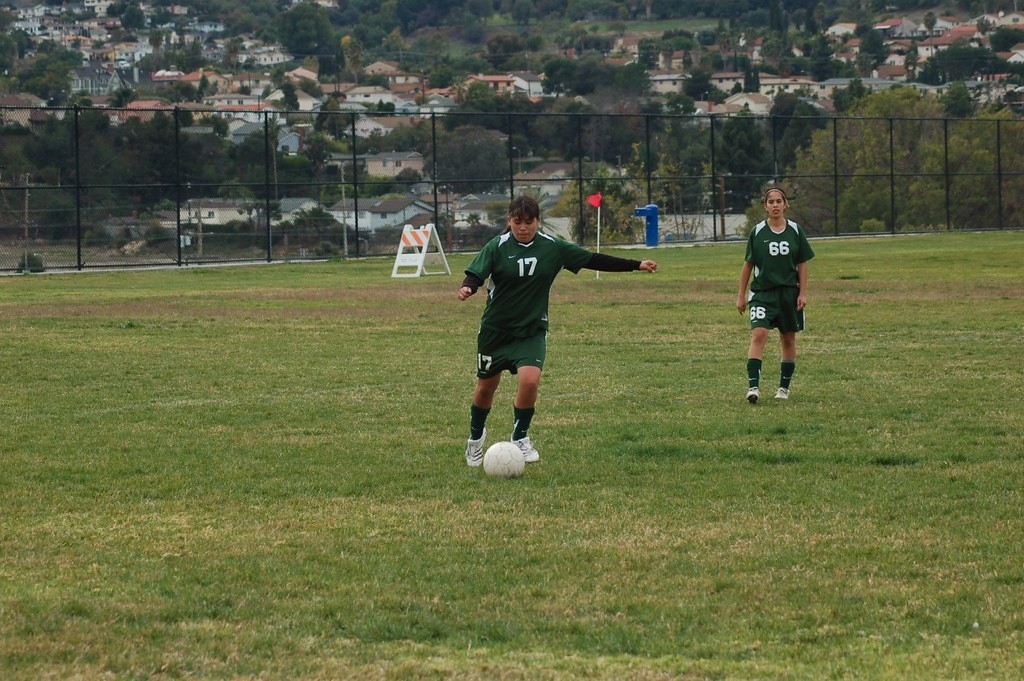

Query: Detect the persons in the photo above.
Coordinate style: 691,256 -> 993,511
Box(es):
458,196 -> 657,467
737,188 -> 815,403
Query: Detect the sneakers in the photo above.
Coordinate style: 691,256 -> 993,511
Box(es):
464,427 -> 486,467
510,433 -> 539,463
746,386 -> 759,404
774,386 -> 790,400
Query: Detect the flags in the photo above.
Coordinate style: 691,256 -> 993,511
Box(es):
587,193 -> 601,207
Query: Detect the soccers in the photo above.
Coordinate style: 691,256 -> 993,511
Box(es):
483,440 -> 526,479
